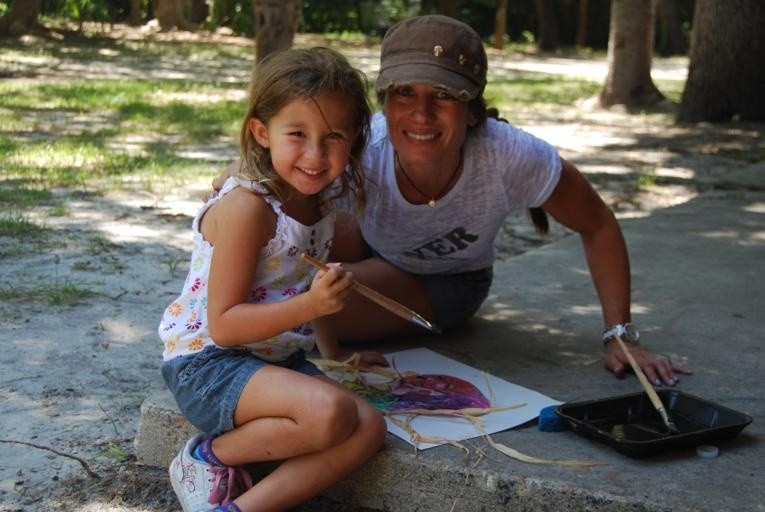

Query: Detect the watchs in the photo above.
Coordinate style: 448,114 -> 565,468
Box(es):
602,322 -> 641,346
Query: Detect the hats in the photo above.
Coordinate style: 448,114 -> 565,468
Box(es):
377,14 -> 488,100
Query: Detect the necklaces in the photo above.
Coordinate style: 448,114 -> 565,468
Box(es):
395,146 -> 462,208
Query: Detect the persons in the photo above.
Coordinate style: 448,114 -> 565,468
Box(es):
204,15 -> 693,387
159,47 -> 387,512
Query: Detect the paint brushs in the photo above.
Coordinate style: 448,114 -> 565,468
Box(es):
612,331 -> 681,434
300,251 -> 445,336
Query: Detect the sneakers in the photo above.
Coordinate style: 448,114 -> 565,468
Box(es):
168,432 -> 239,512
